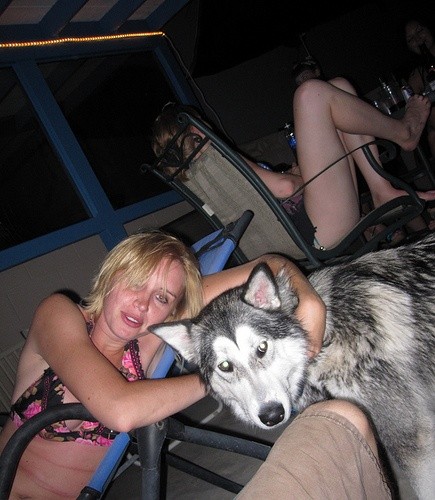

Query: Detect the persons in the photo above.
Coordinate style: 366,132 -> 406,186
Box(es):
404,18 -> 435,156
231,400 -> 392,500
0,233 -> 327,500
290,56 -> 324,85
151,78 -> 435,264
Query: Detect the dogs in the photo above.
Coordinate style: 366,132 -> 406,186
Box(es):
152,128 -> 195,182
147,236 -> 434,500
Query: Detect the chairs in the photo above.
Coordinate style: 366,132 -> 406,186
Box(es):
139,112 -> 435,276
0,210 -> 273,500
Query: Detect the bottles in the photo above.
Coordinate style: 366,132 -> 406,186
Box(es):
373,74 -> 415,111
283,122 -> 298,164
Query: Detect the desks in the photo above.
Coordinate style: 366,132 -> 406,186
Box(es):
389,91 -> 435,192
